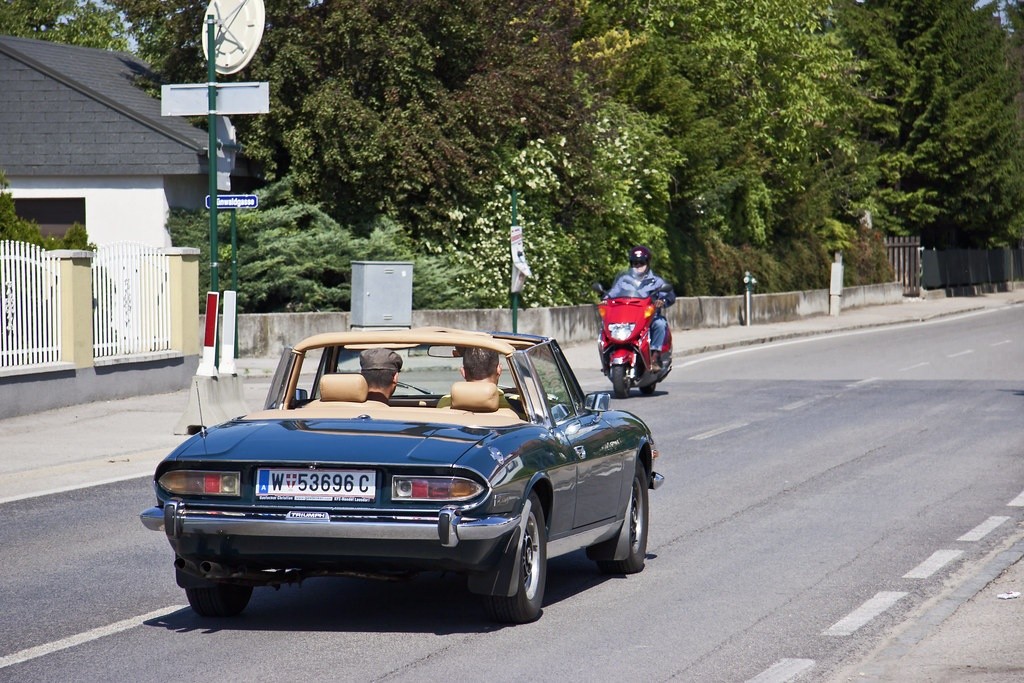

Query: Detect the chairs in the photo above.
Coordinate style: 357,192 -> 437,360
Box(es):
443,381 -> 519,417
308,373 -> 388,407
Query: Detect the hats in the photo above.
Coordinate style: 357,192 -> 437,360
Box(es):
359,347 -> 403,373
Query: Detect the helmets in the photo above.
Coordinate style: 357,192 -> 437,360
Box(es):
629,246 -> 652,264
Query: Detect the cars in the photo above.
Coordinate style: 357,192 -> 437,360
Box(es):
137,326 -> 667,628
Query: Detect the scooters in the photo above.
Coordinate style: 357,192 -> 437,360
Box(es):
590,278 -> 674,400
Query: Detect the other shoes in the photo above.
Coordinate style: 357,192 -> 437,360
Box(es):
651,360 -> 668,374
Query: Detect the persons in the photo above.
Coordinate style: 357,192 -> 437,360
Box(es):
436,347 -> 528,421
358,348 -> 402,407
596,246 -> 676,373
